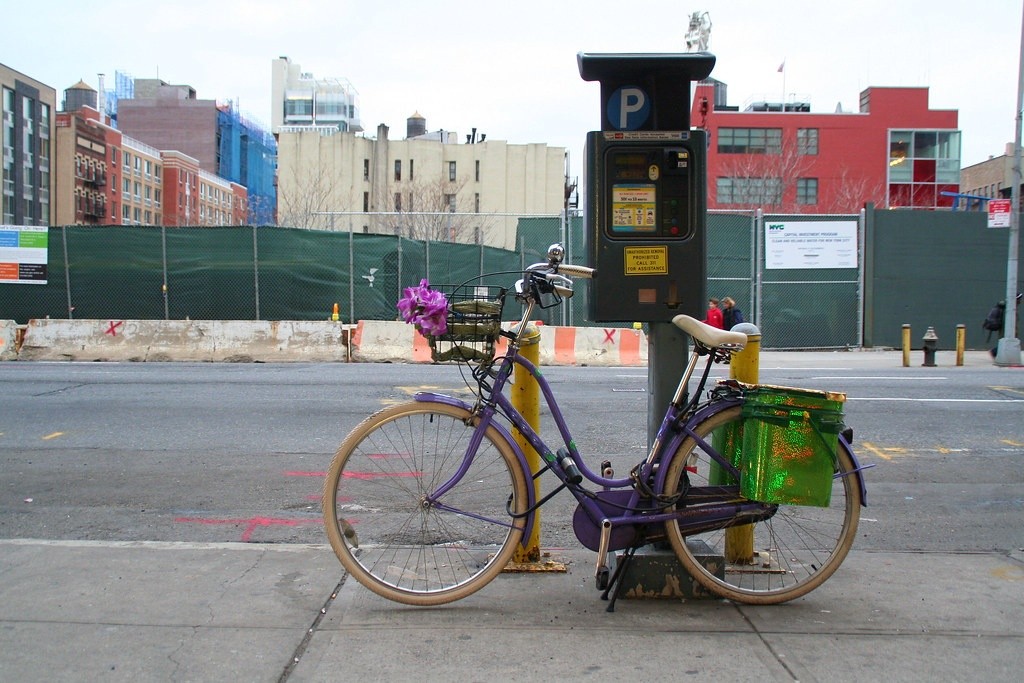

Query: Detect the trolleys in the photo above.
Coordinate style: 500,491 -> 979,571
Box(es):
983,292 -> 1022,358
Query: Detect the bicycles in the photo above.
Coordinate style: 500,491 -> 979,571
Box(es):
321,246 -> 869,604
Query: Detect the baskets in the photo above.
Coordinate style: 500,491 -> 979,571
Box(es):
425,284 -> 515,362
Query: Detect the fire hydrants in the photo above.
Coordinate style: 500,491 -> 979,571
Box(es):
921,325 -> 940,368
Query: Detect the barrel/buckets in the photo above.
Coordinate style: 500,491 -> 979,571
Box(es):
740,384 -> 848,509
707,380 -> 747,485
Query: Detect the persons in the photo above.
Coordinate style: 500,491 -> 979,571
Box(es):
991,290 -> 1023,359
701,298 -> 723,361
721,296 -> 743,364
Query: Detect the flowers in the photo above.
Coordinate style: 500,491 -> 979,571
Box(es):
396,278 -> 450,337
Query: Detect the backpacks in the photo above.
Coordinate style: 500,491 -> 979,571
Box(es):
978,292 -> 1022,342
